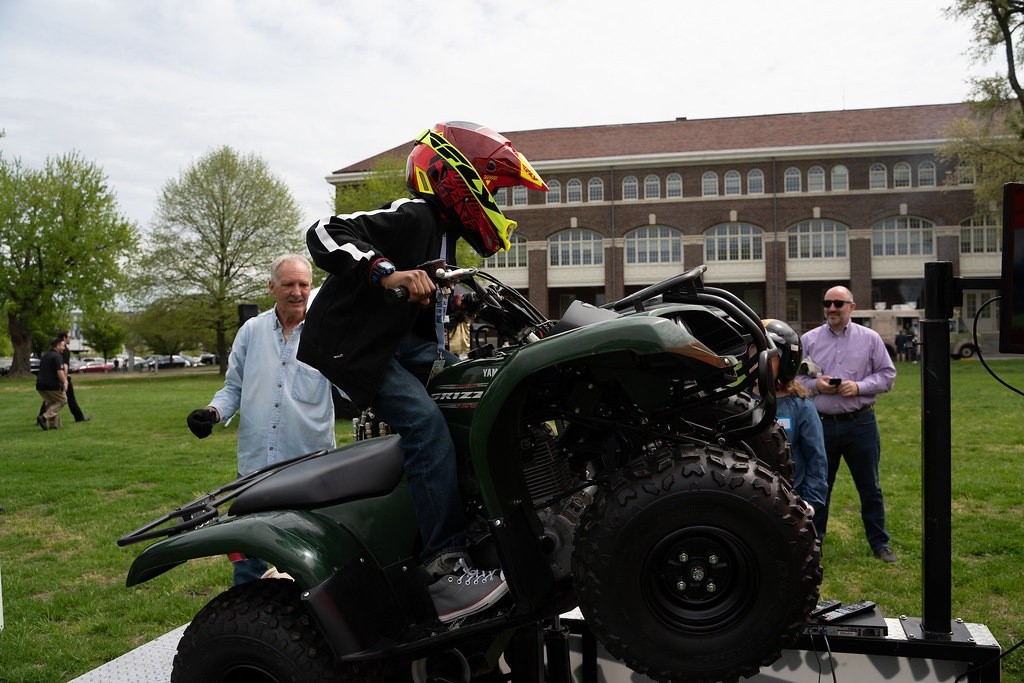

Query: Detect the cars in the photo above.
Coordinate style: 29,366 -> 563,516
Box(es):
0,351 -> 218,375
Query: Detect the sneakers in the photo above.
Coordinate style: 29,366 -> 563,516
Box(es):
876,547 -> 897,562
428,558 -> 508,624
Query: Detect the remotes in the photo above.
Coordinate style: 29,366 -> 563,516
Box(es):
818,600 -> 876,623
809,598 -> 842,618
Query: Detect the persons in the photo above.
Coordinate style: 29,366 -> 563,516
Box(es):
911,334 -> 918,363
297,121 -> 549,623
749,319 -> 829,505
114,359 -> 119,371
895,331 -> 906,361
188,252 -> 351,586
795,286 -> 898,563
36,329 -> 93,431
123,360 -> 127,368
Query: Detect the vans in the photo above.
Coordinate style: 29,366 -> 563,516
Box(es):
850,308 -> 984,361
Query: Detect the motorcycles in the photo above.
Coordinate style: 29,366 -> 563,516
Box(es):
113,260 -> 826,683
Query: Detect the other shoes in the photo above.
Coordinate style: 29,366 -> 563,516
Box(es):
83,417 -> 91,421
38,415 -> 48,430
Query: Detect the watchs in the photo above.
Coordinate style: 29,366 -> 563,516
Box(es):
371,261 -> 396,285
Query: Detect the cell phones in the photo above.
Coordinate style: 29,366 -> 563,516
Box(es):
829,379 -> 842,389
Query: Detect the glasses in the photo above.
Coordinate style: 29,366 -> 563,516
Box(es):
822,300 -> 852,308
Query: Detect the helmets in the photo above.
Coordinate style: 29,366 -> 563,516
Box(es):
406,121 -> 549,258
743,318 -> 803,380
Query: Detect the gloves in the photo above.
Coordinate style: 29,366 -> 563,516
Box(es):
187,409 -> 217,439
